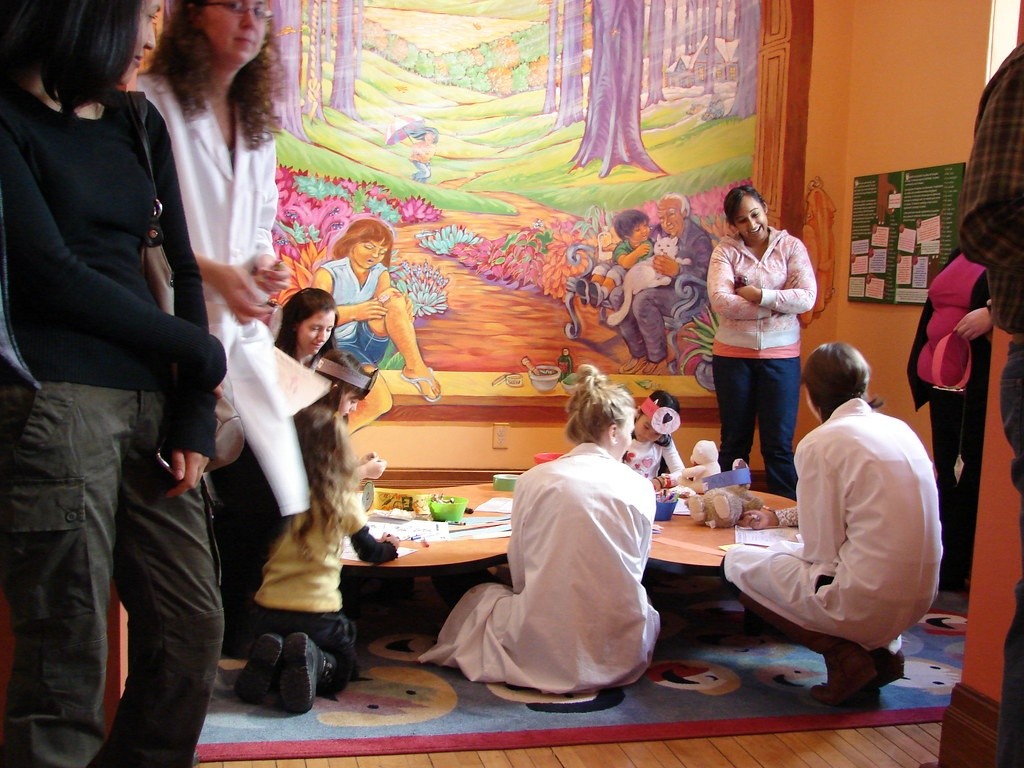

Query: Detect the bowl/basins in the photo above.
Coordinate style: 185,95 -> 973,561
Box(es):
655,494 -> 678,521
429,495 -> 466,524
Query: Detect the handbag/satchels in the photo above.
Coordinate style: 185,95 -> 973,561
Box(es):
128,91 -> 175,318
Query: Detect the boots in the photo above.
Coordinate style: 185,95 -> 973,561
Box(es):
275,631 -> 337,713
863,646 -> 904,690
233,631 -> 286,703
746,599 -> 877,706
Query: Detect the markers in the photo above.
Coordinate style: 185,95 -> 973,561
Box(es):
659,490 -> 681,503
465,508 -> 473,514
411,535 -> 421,540
387,534 -> 391,537
431,493 -> 455,503
448,521 -> 466,525
422,539 -> 429,547
751,514 -> 759,520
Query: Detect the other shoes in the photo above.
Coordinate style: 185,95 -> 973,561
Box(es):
939,566 -> 966,593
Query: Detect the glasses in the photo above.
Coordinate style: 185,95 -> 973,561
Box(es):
197,0 -> 274,22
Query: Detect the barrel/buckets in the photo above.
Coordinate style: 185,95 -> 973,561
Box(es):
535,453 -> 564,465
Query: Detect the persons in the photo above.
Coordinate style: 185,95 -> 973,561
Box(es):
718,343 -> 943,710
416,364 -> 661,694
244,348 -> 379,555
273,286 -> 340,369
905,247 -> 994,593
706,185 -> 817,501
235,405 -> 400,713
126,0 -> 311,700
956,41 -> 1024,768
619,390 -> 689,492
0,0 -> 228,768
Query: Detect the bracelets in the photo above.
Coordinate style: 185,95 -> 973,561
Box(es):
654,477 -> 662,488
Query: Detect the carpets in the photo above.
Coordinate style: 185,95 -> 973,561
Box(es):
193,572 -> 971,762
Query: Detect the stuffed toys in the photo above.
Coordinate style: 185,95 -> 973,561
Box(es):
684,485 -> 763,528
677,440 -> 721,494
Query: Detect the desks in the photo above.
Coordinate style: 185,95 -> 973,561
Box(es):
338,481 -> 798,576
